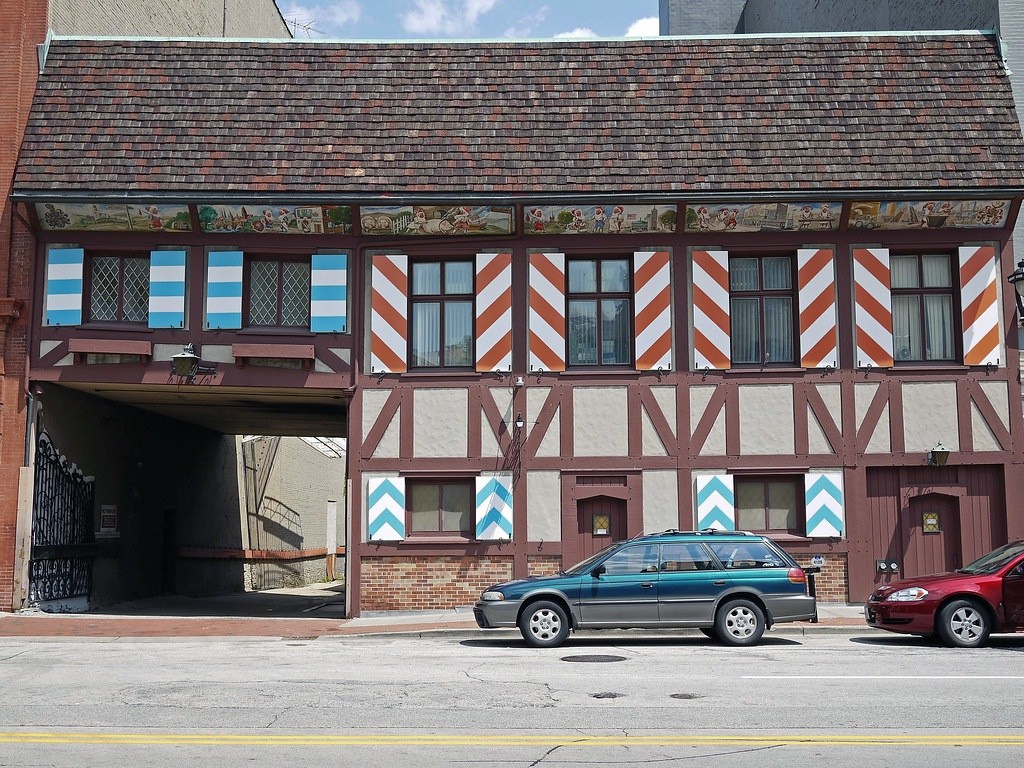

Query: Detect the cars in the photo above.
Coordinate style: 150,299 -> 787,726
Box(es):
863,538 -> 1024,648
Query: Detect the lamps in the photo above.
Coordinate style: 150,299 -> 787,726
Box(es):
1007,258 -> 1024,324
515,413 -> 524,436
928,441 -> 950,472
172,343 -> 216,376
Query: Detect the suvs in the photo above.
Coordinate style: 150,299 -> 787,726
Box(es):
472,527 -> 817,648
